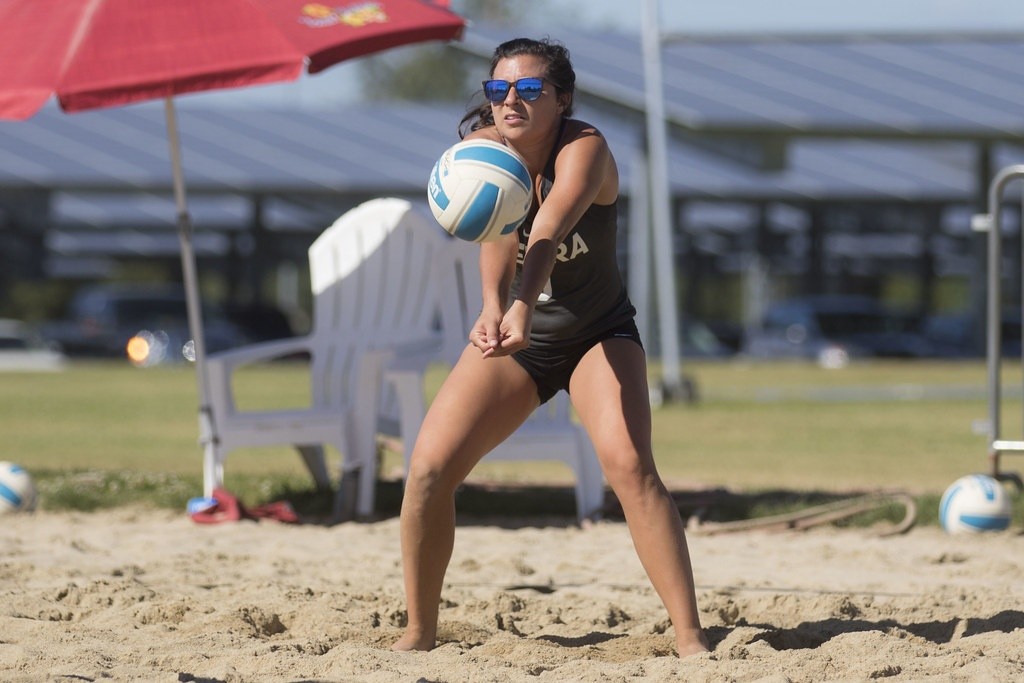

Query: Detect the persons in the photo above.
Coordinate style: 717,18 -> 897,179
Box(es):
395,37 -> 710,660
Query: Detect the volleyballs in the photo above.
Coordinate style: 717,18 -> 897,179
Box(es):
427,137 -> 534,243
938,473 -> 1013,537
0,461 -> 33,514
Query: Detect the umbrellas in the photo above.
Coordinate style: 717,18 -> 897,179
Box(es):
1,0 -> 468,438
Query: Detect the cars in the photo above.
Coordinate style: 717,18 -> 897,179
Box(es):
42,274 -> 306,369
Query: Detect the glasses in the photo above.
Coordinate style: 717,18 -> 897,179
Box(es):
482,76 -> 566,102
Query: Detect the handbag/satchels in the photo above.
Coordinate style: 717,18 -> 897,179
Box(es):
191,488 -> 297,523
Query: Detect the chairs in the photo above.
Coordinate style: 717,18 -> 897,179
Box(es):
202,193 -> 606,525
